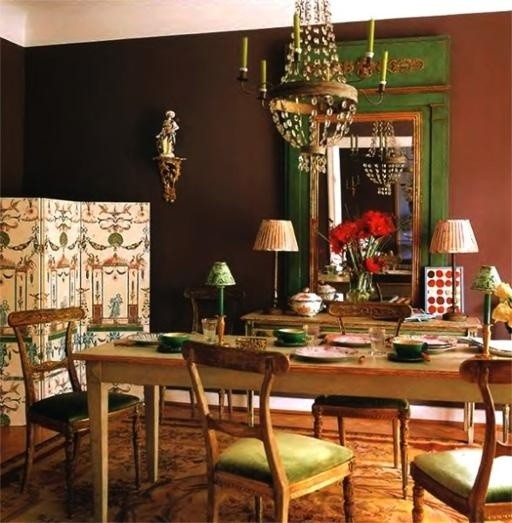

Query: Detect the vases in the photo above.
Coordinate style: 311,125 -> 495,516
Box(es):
344,269 -> 371,305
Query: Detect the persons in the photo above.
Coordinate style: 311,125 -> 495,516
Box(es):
156,110 -> 180,157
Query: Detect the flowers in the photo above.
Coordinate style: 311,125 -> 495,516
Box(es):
492,279 -> 512,330
316,210 -> 398,276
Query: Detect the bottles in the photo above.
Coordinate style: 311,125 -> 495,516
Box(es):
286,287 -> 324,316
317,280 -> 336,301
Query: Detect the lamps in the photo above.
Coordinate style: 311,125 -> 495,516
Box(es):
252,215 -> 300,315
233,2 -> 387,175
346,118 -> 409,195
430,217 -> 478,318
471,260 -> 503,356
204,260 -> 237,342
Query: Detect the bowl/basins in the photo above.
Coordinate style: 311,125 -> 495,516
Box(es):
235,338 -> 267,352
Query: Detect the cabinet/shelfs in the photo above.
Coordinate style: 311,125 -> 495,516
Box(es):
237,304 -> 482,447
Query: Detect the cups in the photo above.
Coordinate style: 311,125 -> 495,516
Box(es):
392,339 -> 428,356
277,328 -> 307,343
201,318 -> 218,344
157,333 -> 191,347
303,322 -> 320,344
367,326 -> 386,357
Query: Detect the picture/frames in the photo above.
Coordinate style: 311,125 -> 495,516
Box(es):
424,265 -> 466,319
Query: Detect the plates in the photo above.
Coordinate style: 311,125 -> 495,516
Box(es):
293,345 -> 360,362
157,346 -> 182,352
126,332 -> 160,343
273,339 -> 308,348
390,335 -> 457,353
387,354 -> 424,362
324,332 -> 389,346
477,343 -> 511,356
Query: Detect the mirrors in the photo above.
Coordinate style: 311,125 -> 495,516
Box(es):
305,107 -> 423,309
323,134 -> 413,277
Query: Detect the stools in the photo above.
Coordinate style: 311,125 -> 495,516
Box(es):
463,398 -> 510,447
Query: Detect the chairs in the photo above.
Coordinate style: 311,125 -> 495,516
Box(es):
179,337 -> 359,523
309,294 -> 416,498
410,352 -> 511,522
8,307 -> 147,521
184,285 -> 247,421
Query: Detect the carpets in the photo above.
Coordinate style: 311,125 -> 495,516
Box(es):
1,401 -> 511,521
